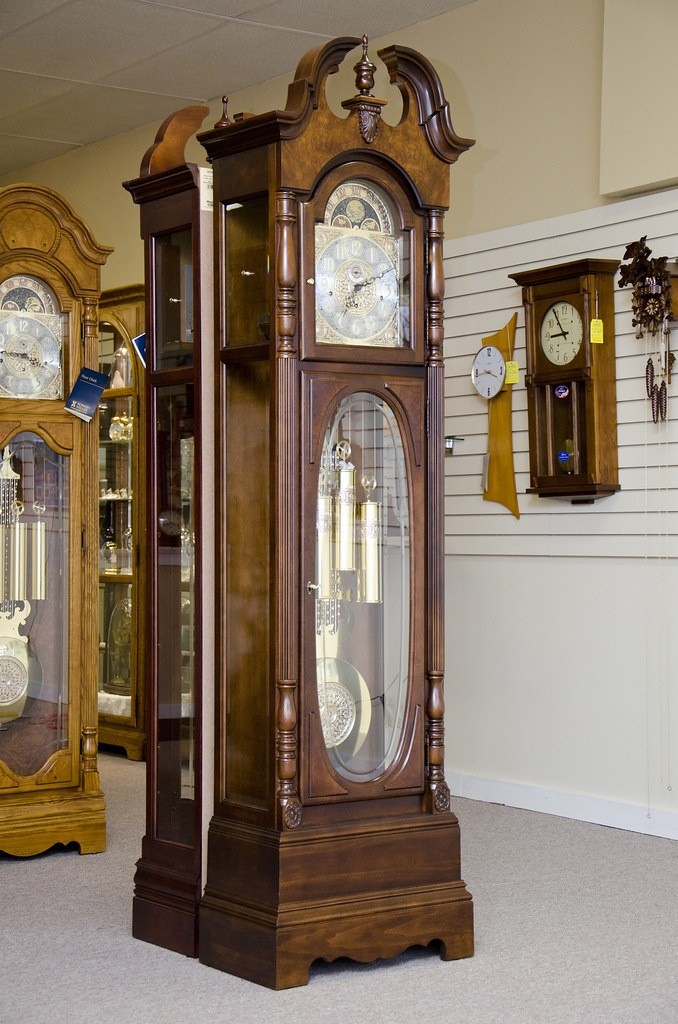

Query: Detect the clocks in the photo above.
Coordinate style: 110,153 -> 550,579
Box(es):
158,508 -> 183,537
468,345 -> 506,399
507,255 -> 624,500
0,184 -> 114,856
121,28 -> 477,993
